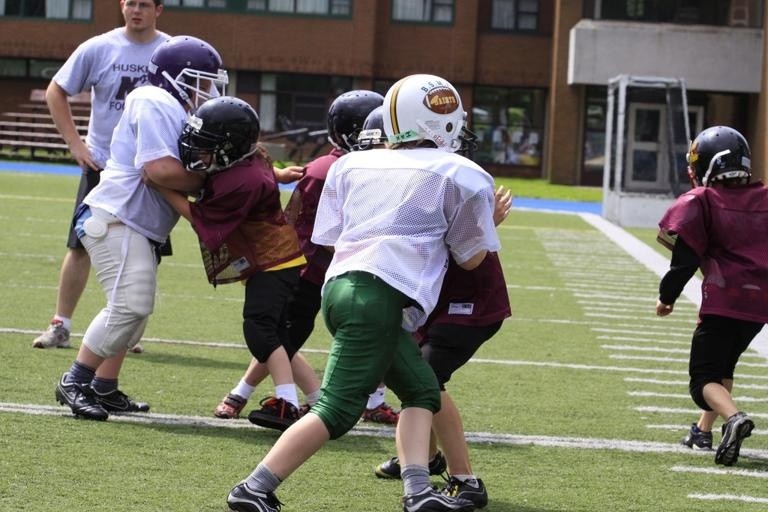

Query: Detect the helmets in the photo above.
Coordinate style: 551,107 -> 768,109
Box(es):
325,89 -> 385,153
381,73 -> 478,155
146,34 -> 229,113
176,96 -> 261,173
356,104 -> 389,150
687,124 -> 753,186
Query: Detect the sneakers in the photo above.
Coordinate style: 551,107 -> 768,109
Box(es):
90,384 -> 150,413
400,485 -> 474,512
680,422 -> 714,451
439,469 -> 489,509
32,319 -> 71,348
374,449 -> 447,479
714,411 -> 755,467
224,480 -> 285,512
213,390 -> 248,420
248,395 -> 300,432
54,371 -> 109,422
362,403 -> 401,425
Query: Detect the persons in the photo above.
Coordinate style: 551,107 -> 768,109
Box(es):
141,95 -> 322,431
54,34 -> 306,422
657,126 -> 763,463
31,0 -> 219,353
357,106 -> 512,510
213,90 -> 399,424
227,74 -> 513,512
